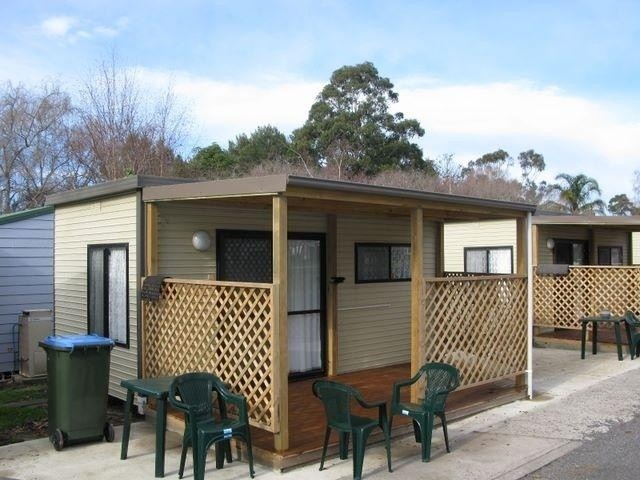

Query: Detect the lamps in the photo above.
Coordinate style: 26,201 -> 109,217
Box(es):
192,229 -> 213,251
547,238 -> 555,250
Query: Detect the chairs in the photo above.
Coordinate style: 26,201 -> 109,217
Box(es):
624,310 -> 640,360
169,372 -> 255,480
311,362 -> 460,480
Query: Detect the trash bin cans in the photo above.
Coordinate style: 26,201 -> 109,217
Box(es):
38,334 -> 115,451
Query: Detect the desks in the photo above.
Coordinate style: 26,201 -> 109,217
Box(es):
579,315 -> 634,361
120,373 -> 233,479
